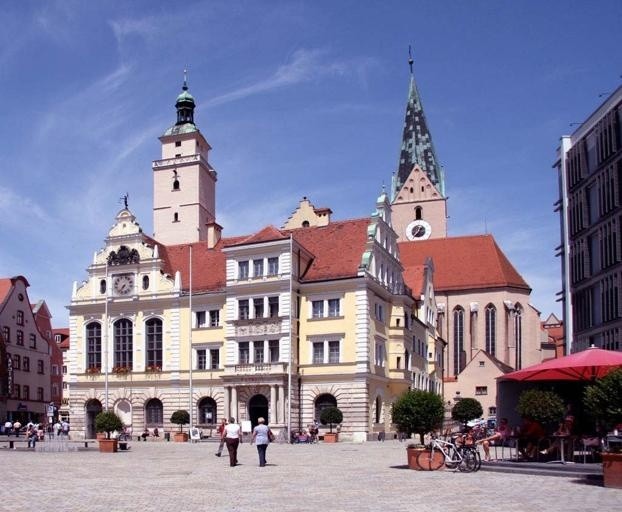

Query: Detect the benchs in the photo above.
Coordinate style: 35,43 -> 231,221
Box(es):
0,440 -> 96,449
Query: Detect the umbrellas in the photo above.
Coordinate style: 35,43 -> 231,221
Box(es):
494,344 -> 622,388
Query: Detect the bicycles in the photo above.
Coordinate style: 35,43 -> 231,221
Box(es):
415,433 -> 484,474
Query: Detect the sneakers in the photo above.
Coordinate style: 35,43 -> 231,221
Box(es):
484,456 -> 491,461
216,453 -> 221,457
540,449 -> 548,455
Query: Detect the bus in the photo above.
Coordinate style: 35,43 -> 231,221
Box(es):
487,415 -> 495,438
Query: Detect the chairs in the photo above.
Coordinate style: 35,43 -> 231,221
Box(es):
489,415 -> 618,465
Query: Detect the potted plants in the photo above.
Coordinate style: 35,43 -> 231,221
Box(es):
170,409 -> 191,442
94,412 -> 124,452
390,387 -> 446,470
582,367 -> 622,490
319,406 -> 343,443
450,398 -> 483,445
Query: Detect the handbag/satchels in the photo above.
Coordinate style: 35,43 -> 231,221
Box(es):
268,430 -> 275,442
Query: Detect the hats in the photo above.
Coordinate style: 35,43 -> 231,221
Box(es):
566,416 -> 574,422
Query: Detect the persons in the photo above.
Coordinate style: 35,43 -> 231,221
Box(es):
52,419 -> 70,438
120,192 -> 128,209
222,417 -> 241,467
4,418 -> 44,448
290,424 -> 320,445
446,415 -> 621,462
153,427 -> 161,437
215,419 -> 228,457
250,417 -> 273,467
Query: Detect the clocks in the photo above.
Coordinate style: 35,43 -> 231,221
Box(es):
113,273 -> 135,297
405,219 -> 432,241
